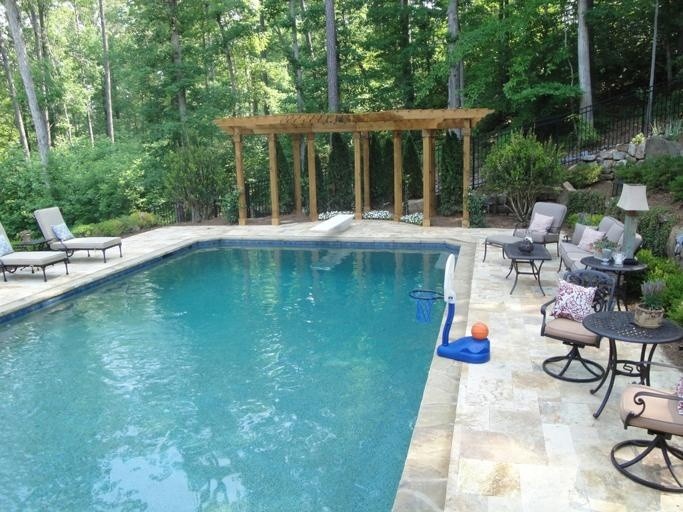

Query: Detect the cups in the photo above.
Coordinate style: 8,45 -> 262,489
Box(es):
601,248 -> 626,268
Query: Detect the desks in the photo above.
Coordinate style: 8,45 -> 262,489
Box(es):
580,302 -> 683,419
501,241 -> 552,298
12,238 -> 54,273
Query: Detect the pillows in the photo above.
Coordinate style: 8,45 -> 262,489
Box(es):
0,234 -> 14,257
570,222 -> 599,247
577,226 -> 606,255
50,222 -> 74,241
551,278 -> 598,322
528,213 -> 555,235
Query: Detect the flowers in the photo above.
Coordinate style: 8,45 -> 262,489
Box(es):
639,281 -> 670,307
584,233 -> 619,253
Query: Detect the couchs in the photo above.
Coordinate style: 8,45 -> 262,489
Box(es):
556,214 -> 643,288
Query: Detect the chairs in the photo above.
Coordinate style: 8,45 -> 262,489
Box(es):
0,220 -> 69,282
31,204 -> 123,263
481,200 -> 568,261
540,270 -> 614,382
610,357 -> 682,493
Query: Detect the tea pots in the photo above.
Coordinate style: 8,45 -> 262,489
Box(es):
518,237 -> 534,253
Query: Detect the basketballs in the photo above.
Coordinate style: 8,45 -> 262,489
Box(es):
471,322 -> 488,339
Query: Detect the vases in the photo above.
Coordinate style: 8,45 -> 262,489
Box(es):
601,249 -> 612,261
633,305 -> 664,329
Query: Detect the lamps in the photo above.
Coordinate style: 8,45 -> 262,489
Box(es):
614,182 -> 650,263
